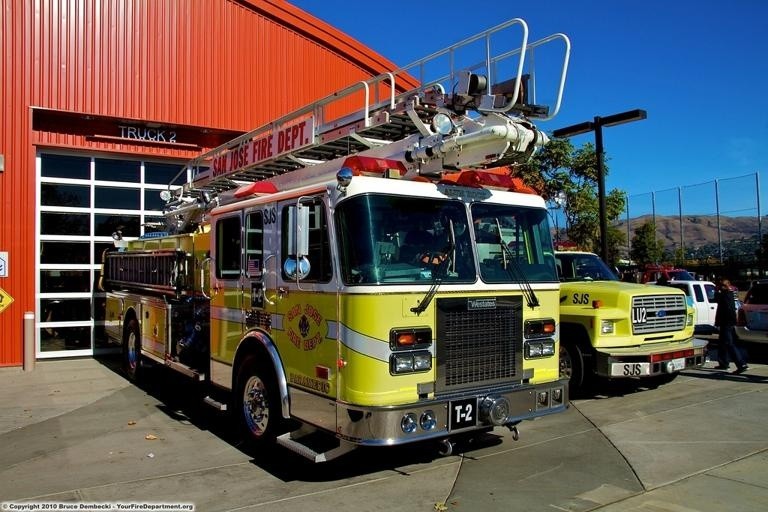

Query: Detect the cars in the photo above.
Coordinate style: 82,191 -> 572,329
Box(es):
734,278 -> 768,345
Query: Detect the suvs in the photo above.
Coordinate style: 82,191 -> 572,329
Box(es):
491,238 -> 709,385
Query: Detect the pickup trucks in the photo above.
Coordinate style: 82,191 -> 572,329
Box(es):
624,262 -> 742,332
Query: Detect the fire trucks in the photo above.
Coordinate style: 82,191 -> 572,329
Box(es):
97,18 -> 571,463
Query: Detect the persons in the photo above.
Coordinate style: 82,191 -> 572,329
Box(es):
713,279 -> 749,374
